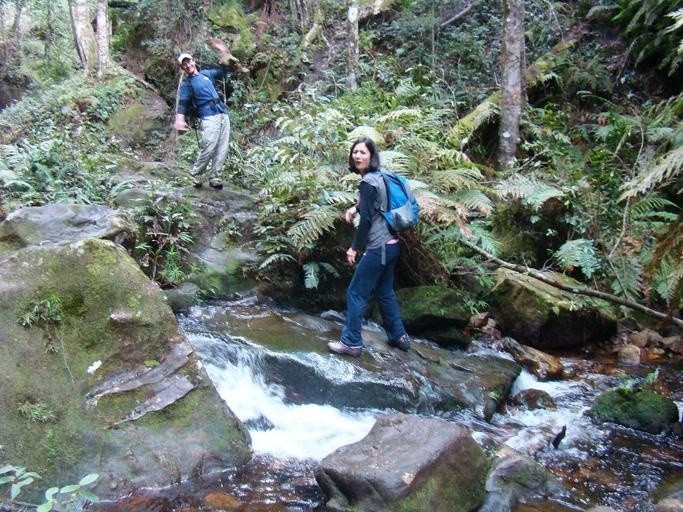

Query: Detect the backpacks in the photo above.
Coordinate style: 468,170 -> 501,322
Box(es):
379,170 -> 420,231
187,73 -> 219,118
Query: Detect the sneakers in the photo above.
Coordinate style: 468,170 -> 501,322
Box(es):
210,179 -> 223,188
192,177 -> 202,187
385,333 -> 411,350
328,339 -> 362,357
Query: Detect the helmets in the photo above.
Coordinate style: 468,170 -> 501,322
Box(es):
178,54 -> 192,66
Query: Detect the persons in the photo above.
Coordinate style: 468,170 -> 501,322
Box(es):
325,135 -> 414,357
172,38 -> 233,190
